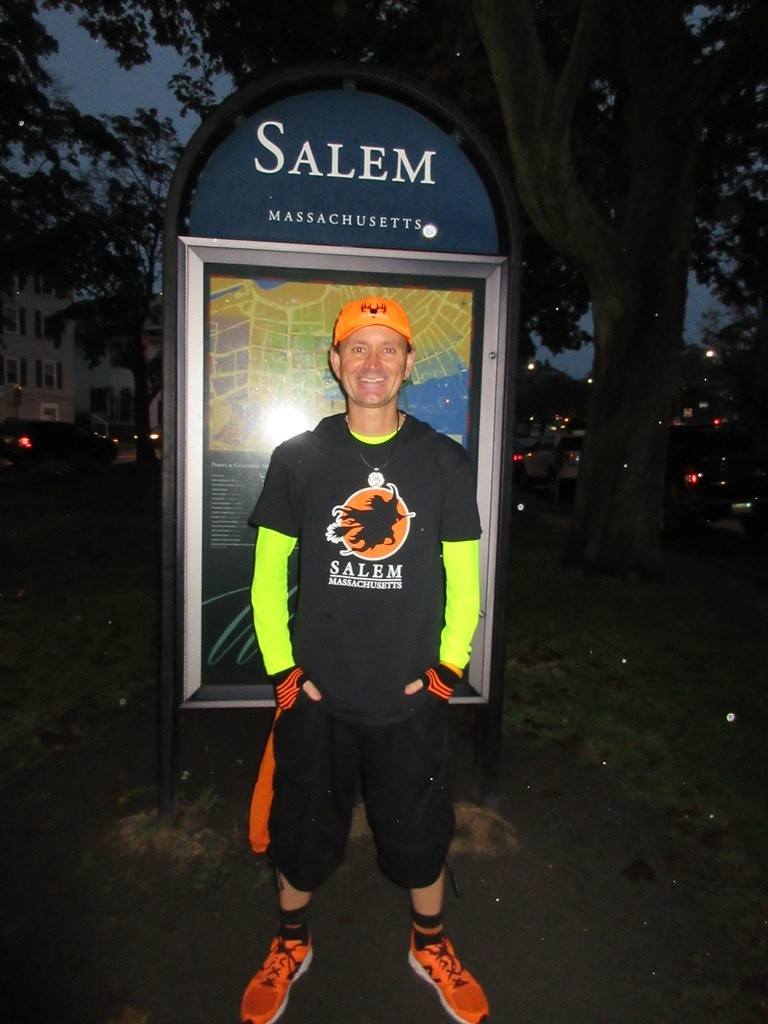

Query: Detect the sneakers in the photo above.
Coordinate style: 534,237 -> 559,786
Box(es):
240,928 -> 314,1024
408,930 -> 490,1024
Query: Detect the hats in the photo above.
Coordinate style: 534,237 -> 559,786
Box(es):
333,296 -> 411,346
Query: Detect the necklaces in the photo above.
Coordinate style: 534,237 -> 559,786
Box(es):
346,412 -> 399,487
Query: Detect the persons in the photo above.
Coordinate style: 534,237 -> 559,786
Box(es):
241,297 -> 491,1024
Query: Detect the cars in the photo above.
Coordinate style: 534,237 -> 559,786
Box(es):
513,436 -> 538,481
0,417 -> 118,465
519,434 -> 585,492
131,424 -> 163,451
666,419 -> 768,538
